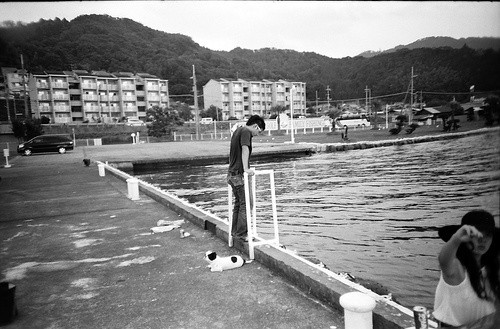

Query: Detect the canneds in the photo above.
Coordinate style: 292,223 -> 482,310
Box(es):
180,229 -> 184,238
413,306 -> 429,329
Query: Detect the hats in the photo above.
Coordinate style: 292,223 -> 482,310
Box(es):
462,209 -> 500,238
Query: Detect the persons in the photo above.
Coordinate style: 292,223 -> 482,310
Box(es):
432,210 -> 500,329
227,115 -> 265,241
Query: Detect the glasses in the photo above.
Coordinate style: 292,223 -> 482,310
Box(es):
256,124 -> 263,135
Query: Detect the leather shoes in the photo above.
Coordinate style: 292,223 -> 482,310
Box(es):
231,233 -> 255,241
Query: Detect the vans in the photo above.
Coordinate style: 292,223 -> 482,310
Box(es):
127,119 -> 144,124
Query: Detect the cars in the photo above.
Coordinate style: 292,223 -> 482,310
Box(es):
16,133 -> 75,156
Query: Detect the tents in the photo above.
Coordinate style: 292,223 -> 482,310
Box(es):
414,106 -> 453,123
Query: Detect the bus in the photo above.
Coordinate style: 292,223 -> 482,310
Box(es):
334,115 -> 371,129
